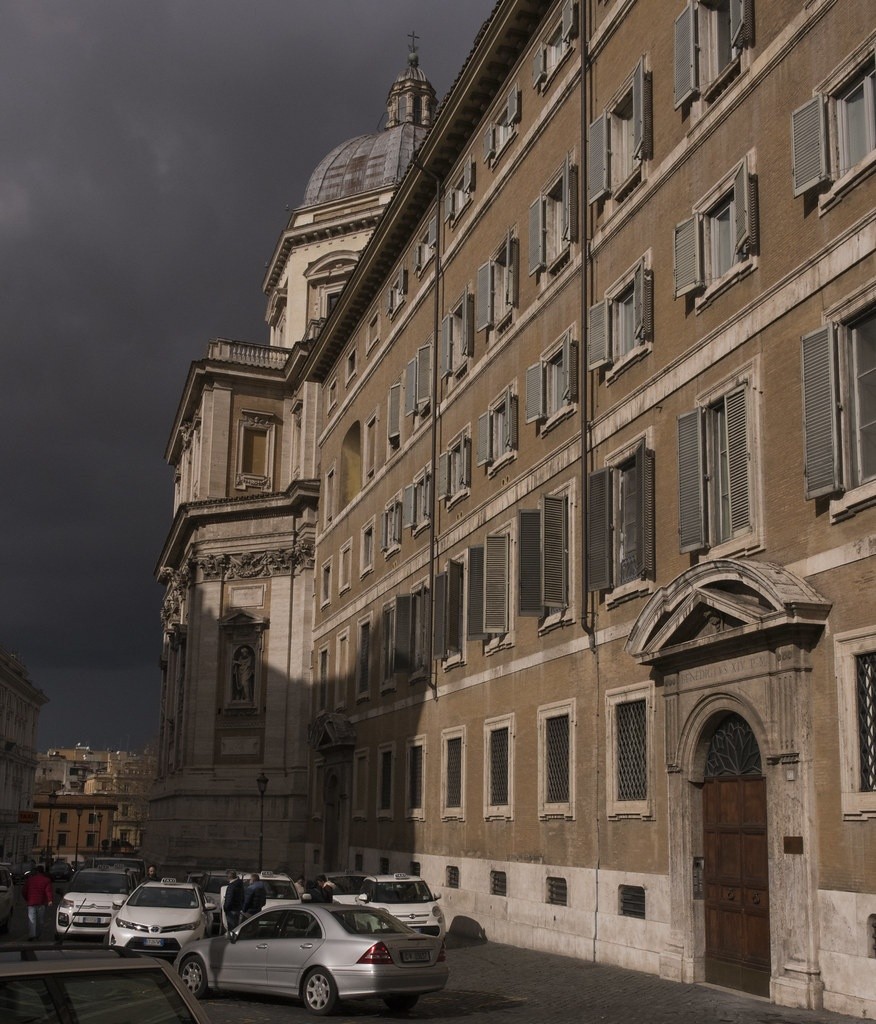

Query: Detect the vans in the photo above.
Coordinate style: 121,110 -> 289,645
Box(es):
83,858 -> 146,883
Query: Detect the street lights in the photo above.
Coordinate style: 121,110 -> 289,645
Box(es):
74,804 -> 83,872
45,789 -> 59,873
254,771 -> 271,873
96,812 -> 103,856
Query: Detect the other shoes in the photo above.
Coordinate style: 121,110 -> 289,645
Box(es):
27,937 -> 34,941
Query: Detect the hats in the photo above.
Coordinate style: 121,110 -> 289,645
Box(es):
35,866 -> 44,871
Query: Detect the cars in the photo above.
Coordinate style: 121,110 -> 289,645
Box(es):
108,877 -> 217,963
185,871 -> 302,936
315,871 -> 368,905
355,871 -> 446,942
10,861 -> 38,885
0,863 -> 15,935
50,862 -> 74,883
169,901 -> 448,1016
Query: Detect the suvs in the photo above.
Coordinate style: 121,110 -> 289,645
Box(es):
55,867 -> 138,942
0,940 -> 212,1024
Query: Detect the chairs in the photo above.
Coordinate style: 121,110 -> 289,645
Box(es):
294,916 -> 309,932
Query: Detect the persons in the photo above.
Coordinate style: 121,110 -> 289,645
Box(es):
292,873 -> 335,902
221,869 -> 267,940
21,865 -> 55,942
232,648 -> 255,702
138,867 -> 163,887
